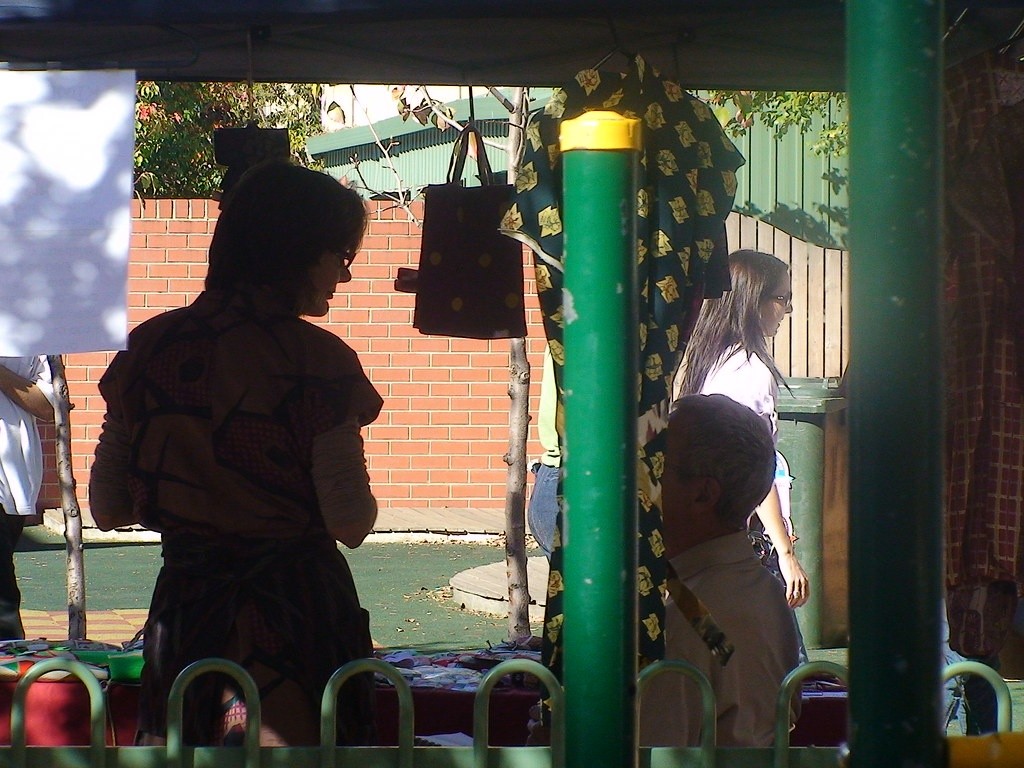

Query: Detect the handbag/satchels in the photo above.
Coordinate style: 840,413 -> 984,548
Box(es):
413,123 -> 527,340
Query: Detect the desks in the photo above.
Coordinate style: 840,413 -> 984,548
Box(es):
0,645 -> 847,747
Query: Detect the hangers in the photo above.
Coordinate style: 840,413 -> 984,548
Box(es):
591,13 -> 634,71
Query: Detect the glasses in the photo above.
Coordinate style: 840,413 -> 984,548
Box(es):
766,290 -> 792,307
323,246 -> 357,269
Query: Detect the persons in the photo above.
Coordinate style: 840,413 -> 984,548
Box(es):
671,249 -> 814,610
640,390 -> 802,745
527,334 -> 562,570
89,158 -> 383,745
0,357 -> 62,635
938,588 -> 1001,738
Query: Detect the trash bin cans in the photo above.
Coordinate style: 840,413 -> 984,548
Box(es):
773,377 -> 846,651
